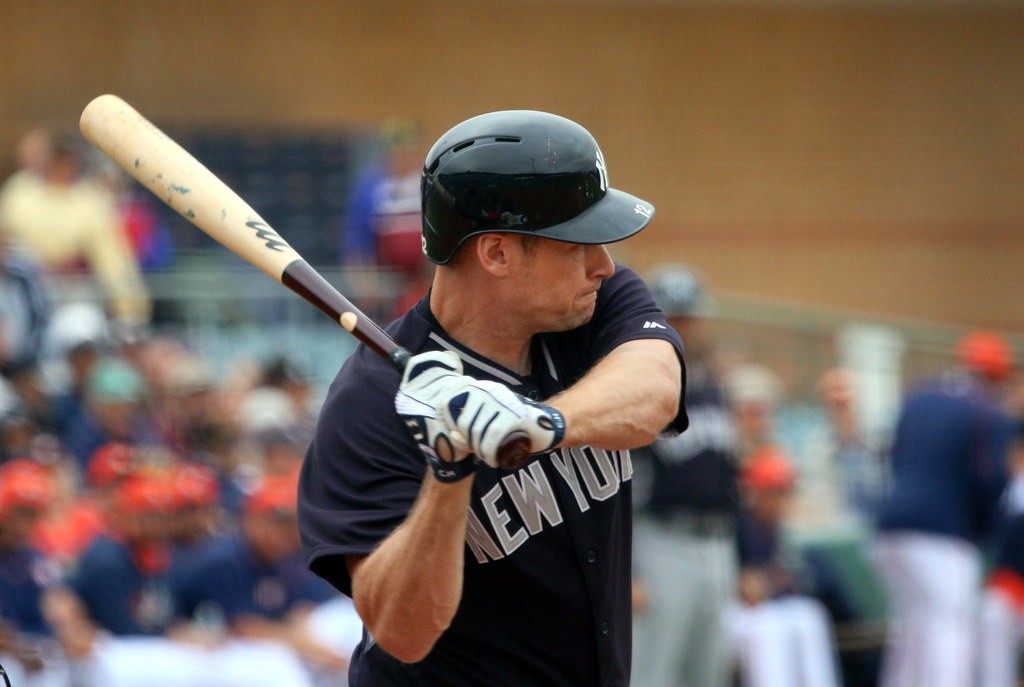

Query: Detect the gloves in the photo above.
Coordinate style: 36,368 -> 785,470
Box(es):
436,376 -> 566,468
394,350 -> 482,483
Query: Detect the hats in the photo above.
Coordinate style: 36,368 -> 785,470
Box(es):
743,449 -> 797,490
722,364 -> 774,401
0,303 -> 304,566
961,335 -> 1013,379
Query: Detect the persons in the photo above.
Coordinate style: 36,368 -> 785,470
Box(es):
0,132 -> 1024,687
296,108 -> 690,687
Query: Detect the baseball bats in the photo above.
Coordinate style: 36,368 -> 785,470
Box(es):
78,91 -> 534,474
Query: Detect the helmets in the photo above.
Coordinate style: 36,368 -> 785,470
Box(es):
420,109 -> 655,264
656,268 -> 704,321
